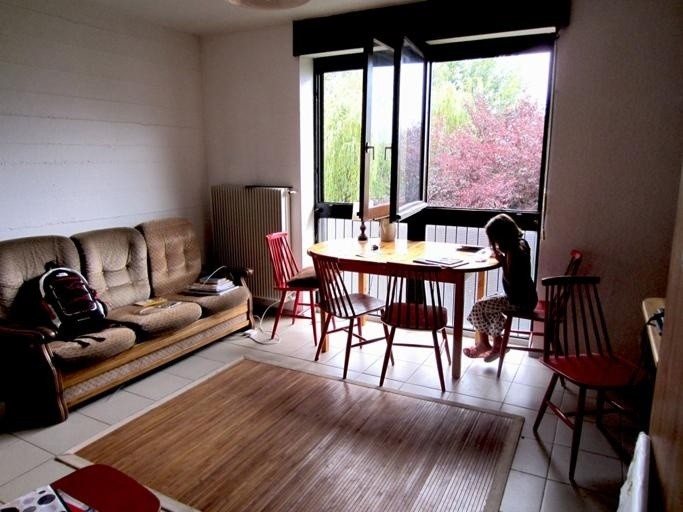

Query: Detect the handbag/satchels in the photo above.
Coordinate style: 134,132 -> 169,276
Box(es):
36,262 -> 107,341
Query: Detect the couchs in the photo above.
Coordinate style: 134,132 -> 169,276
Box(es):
0,218 -> 256,421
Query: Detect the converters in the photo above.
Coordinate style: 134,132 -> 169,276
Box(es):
244,329 -> 257,338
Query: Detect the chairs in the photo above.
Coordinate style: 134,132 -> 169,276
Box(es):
310,251 -> 394,379
533,274 -> 647,479
380,262 -> 450,392
497,249 -> 582,386
266,232 -> 336,346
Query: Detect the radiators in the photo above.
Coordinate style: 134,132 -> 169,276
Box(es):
211,184 -> 296,303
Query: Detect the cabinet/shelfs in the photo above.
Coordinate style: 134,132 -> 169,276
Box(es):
647,171 -> 683,511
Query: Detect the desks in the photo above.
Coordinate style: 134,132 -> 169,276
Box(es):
307,239 -> 506,380
642,298 -> 666,367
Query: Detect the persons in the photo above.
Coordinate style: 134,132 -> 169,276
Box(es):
463,213 -> 539,362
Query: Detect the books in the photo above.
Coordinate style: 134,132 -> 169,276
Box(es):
412,244 -> 497,269
0,484 -> 100,512
134,276 -> 240,313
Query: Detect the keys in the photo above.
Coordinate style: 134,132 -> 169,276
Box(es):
372,244 -> 379,250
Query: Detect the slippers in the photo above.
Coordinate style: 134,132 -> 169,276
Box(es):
464,344 -> 509,362
